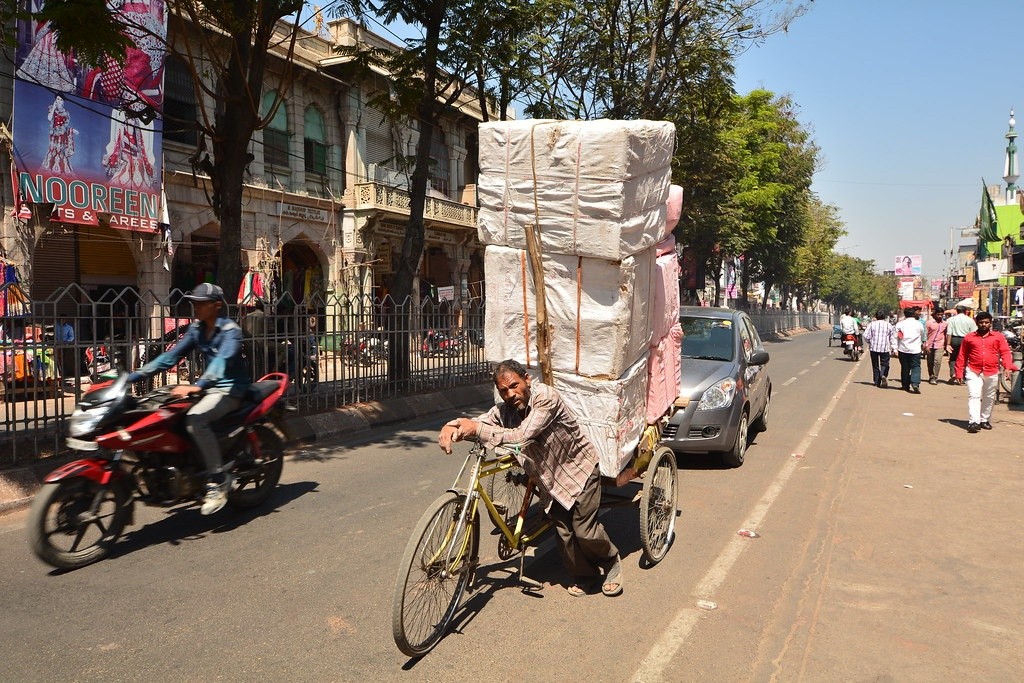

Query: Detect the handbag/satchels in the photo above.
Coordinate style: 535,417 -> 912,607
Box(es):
898,331 -> 903,340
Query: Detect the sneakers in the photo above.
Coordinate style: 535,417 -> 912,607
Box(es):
201,474 -> 232,515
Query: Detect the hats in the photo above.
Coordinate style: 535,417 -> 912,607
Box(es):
183,283 -> 224,302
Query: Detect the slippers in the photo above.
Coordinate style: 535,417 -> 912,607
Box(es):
568,574 -> 600,597
601,554 -> 624,595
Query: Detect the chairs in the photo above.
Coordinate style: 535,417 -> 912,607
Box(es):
703,327 -> 732,360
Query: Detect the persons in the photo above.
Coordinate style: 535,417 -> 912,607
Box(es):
901,255 -> 911,272
955,312 -> 1014,434
101,109 -> 158,194
837,302 -> 977,393
39,92 -> 80,176
56,313 -> 74,345
439,360 -> 624,598
16,0 -> 168,112
115,282 -> 250,516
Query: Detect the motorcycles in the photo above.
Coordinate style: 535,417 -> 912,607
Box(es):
82,334 -> 125,385
26,370 -> 302,571
338,322 -> 389,368
842,328 -> 865,363
420,326 -> 467,358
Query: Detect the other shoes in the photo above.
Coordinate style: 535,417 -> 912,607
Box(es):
961,379 -> 966,384
912,383 -> 921,394
929,375 -> 938,385
901,385 -> 910,390
875,382 -> 880,387
948,375 -> 956,383
980,422 -> 992,429
968,423 -> 981,433
881,375 -> 887,387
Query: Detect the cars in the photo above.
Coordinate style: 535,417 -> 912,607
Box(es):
656,305 -> 774,467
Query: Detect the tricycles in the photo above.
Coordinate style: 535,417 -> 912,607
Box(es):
390,391 -> 689,657
828,323 -> 842,347
